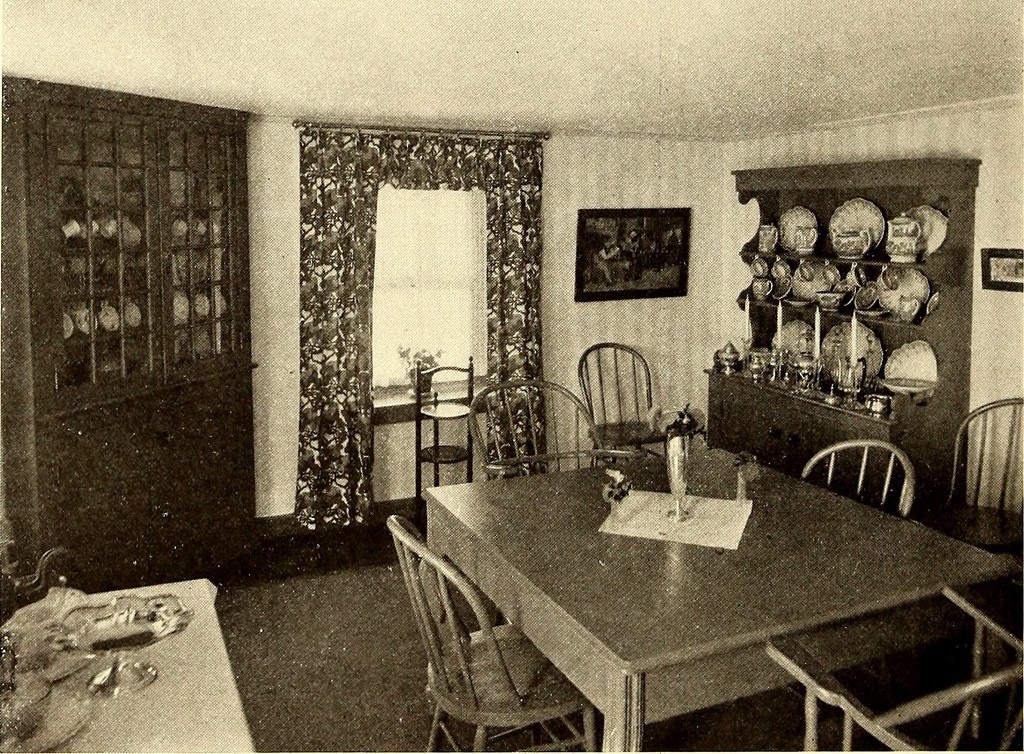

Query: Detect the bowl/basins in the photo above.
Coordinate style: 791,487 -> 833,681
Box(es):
817,293 -> 845,311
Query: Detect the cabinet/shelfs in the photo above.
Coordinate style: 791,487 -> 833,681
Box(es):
2,75 -> 258,624
703,160 -> 982,528
415,355 -> 474,531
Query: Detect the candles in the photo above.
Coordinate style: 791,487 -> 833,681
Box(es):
800,306 -> 827,360
841,309 -> 868,366
765,300 -> 791,357
739,292 -> 756,344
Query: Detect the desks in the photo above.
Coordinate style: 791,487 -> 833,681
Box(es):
2,579 -> 260,754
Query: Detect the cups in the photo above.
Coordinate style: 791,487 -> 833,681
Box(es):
771,255 -> 791,279
750,255 -> 768,276
63,213 -> 207,240
846,261 -> 866,287
795,257 -> 814,282
821,260 -> 840,285
753,279 -> 773,300
878,265 -> 898,290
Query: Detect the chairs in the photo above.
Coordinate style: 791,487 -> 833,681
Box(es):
469,382 -> 645,627
766,587 -> 1023,750
387,514 -> 598,754
800,437 -> 917,519
578,343 -> 667,468
927,399 -> 1023,547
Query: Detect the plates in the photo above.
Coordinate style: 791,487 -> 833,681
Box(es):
46,594 -> 193,655
39,650 -> 97,682
63,293 -> 225,338
885,342 -> 937,398
821,322 -> 882,387
87,663 -> 158,694
27,714 -> 92,751
881,379 -> 934,394
773,197 -> 947,314
0,674 -> 52,708
772,320 -> 814,373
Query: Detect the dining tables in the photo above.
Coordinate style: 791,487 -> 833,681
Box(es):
420,448 -> 1022,753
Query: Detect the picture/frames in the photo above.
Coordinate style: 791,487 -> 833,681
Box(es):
981,249 -> 1024,293
575,209 -> 692,303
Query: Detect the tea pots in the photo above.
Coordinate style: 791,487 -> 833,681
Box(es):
894,294 -> 920,323
759,225 -> 778,252
886,212 -> 921,263
795,224 -> 818,254
828,225 -> 871,259
